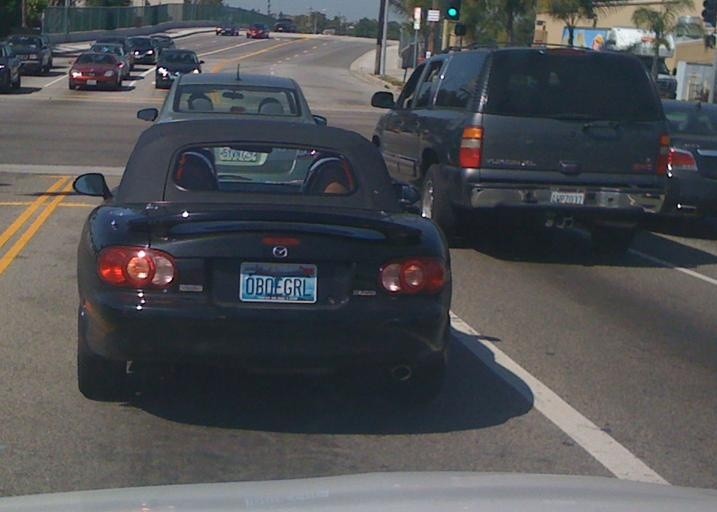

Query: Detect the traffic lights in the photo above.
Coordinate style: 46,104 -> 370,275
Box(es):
455,25 -> 466,35
445,0 -> 460,20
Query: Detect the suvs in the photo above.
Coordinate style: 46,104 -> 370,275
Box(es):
364,43 -> 667,262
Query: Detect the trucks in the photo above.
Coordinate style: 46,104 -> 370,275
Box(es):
560,25 -> 676,79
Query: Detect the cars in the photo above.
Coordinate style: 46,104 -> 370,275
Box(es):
0,29 -> 200,94
274,18 -> 297,32
216,23 -> 240,36
658,97 -> 717,232
137,63 -> 326,126
247,23 -> 270,39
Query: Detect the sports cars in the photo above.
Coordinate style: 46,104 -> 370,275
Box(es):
70,116 -> 455,406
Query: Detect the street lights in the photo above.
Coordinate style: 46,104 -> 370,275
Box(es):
307,4 -> 327,34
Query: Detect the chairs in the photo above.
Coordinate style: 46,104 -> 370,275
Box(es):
192,98 -> 213,111
259,101 -> 284,115
